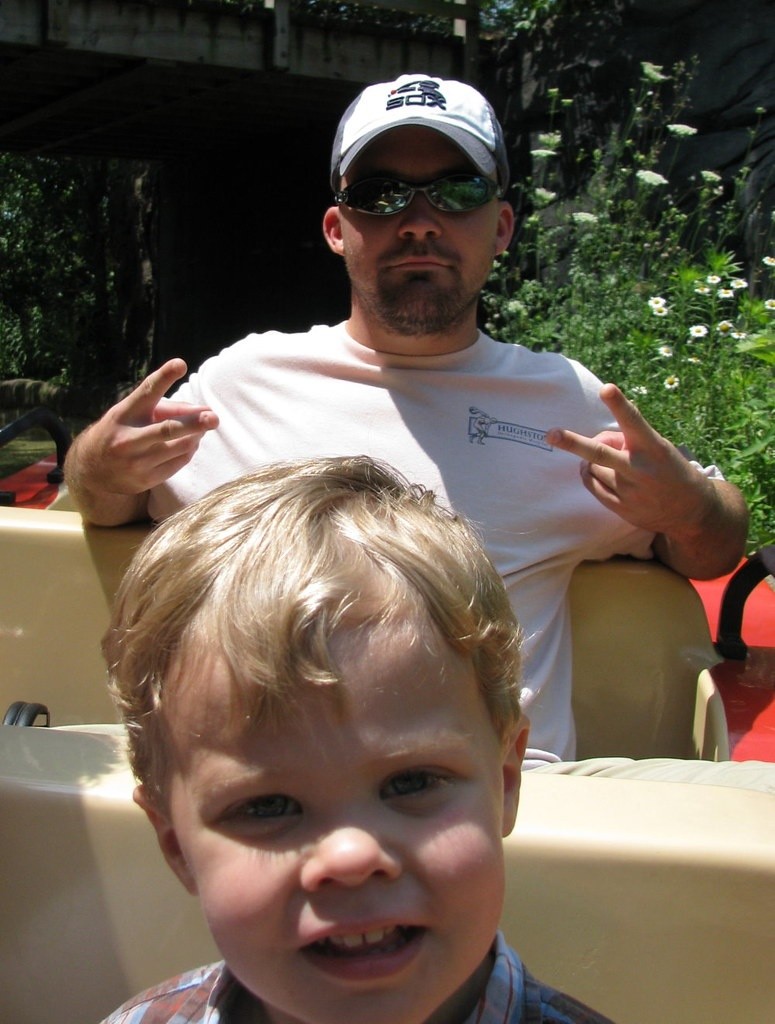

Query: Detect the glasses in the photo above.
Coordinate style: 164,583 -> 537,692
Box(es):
334,171 -> 502,216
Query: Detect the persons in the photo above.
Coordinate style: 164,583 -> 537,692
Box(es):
97,459 -> 617,1024
64,74 -> 750,801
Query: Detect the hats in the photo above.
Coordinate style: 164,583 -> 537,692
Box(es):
331,74 -> 509,197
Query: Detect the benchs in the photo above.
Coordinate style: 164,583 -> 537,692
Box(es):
0,504 -> 775,1024
